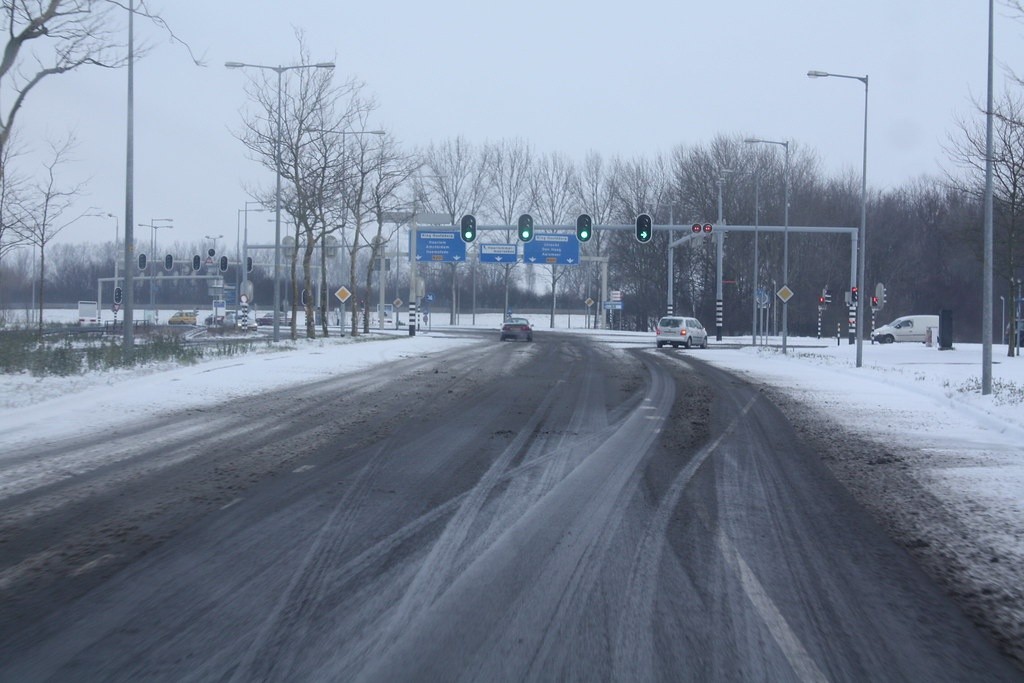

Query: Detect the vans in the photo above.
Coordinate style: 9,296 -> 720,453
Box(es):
870,315 -> 939,344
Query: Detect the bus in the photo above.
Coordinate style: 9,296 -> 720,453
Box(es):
1002,298 -> 1024,347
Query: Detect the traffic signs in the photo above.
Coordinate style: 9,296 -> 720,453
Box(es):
478,234 -> 579,266
408,228 -> 465,263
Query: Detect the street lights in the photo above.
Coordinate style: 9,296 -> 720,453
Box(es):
743,139 -> 791,354
382,172 -> 447,335
720,169 -> 756,347
808,68 -> 870,368
1000,279 -> 1022,357
223,55 -> 388,340
106,200 -> 271,330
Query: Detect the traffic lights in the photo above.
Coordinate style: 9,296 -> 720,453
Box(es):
578,215 -> 591,242
247,258 -> 254,274
824,289 -> 832,303
520,215 -> 533,241
852,287 -> 859,302
137,253 -> 147,269
192,255 -> 200,271
872,296 -> 879,306
114,287 -> 123,304
638,215 -> 652,242
462,215 -> 476,242
164,254 -> 174,270
883,288 -> 887,304
220,256 -> 228,272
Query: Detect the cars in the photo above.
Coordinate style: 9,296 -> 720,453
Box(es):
500,318 -> 535,343
656,316 -> 709,349
167,311 -> 297,332
